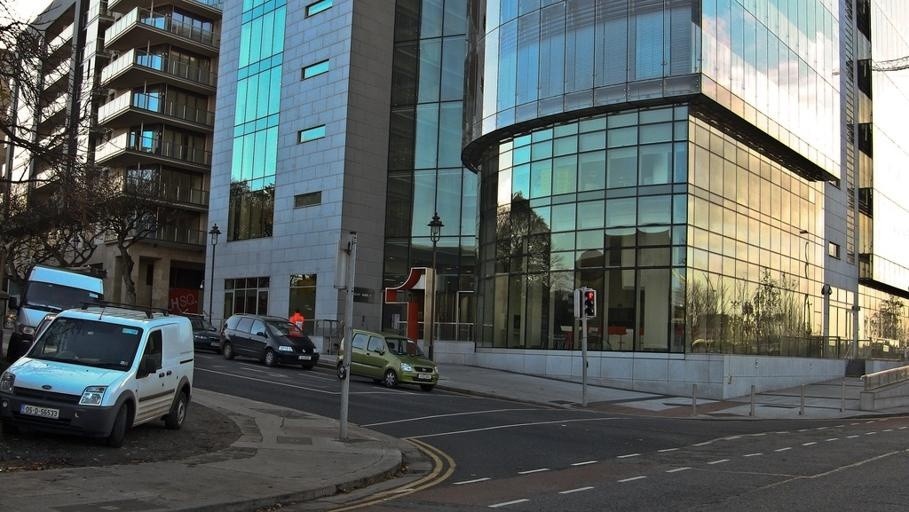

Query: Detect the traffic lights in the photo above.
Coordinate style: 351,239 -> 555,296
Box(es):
566,287 -> 580,319
579,288 -> 597,319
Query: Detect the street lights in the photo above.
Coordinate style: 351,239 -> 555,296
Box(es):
427,210 -> 444,361
207,221 -> 221,326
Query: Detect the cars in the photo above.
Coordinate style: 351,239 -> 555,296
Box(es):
336,327 -> 439,392
216,311 -> 320,370
178,310 -> 221,355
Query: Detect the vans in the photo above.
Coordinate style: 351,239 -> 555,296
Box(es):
0,297 -> 196,449
4,263 -> 106,360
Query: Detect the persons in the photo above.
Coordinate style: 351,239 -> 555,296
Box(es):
288,305 -> 305,337
